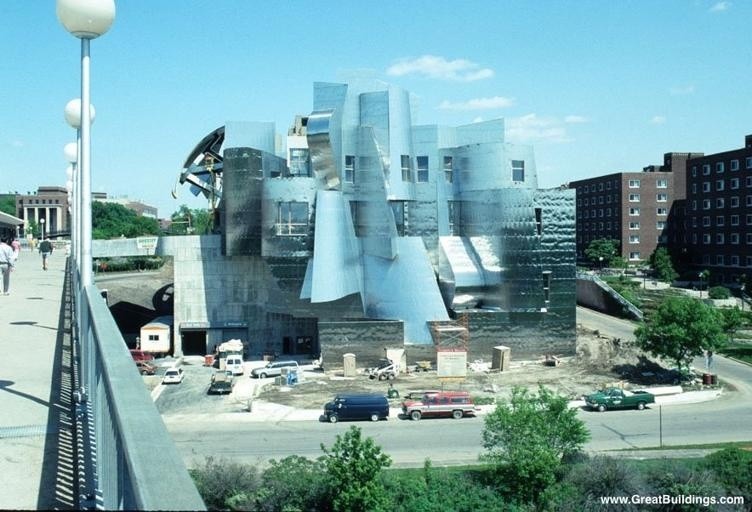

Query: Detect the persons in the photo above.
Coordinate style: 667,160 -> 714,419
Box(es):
0,230 -> 71,296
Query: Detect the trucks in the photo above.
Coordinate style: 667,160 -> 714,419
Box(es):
223,354 -> 244,376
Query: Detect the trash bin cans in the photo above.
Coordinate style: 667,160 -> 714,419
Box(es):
275,377 -> 280,386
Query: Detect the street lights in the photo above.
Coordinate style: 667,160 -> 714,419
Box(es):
61,91 -> 96,267
636,266 -> 656,288
16,225 -> 21,239
598,254 -> 603,276
51,0 -> 119,286
699,270 -> 704,297
38,218 -> 46,241
741,282 -> 747,311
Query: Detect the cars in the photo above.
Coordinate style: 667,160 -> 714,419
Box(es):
161,367 -> 184,385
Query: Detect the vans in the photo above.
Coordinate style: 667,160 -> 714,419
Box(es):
318,393 -> 390,425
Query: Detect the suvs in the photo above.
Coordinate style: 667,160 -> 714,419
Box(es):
133,359 -> 158,376
129,349 -> 153,362
399,390 -> 477,423
250,359 -> 299,378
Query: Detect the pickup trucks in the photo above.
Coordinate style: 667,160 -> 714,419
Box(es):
583,384 -> 656,414
207,369 -> 234,394
